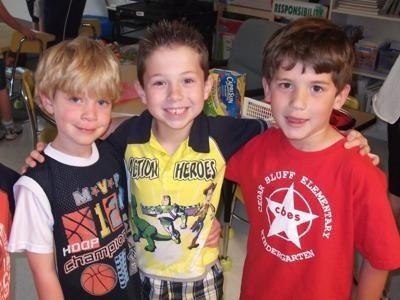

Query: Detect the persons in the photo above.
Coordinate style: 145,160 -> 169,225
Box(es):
224,17 -> 400,300
21,18 -> 381,300
6,36 -> 222,300
0,0 -> 39,140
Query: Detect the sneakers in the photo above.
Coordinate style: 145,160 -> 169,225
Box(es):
6,127 -> 22,140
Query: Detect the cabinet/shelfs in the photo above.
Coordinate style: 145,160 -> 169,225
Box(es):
216,0 -> 400,141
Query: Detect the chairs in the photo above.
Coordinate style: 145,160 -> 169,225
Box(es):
5,29 -> 56,95
21,70 -> 59,149
222,18 -> 287,100
77,19 -> 102,41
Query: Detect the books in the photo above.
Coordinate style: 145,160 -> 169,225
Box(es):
332,0 -> 400,77
